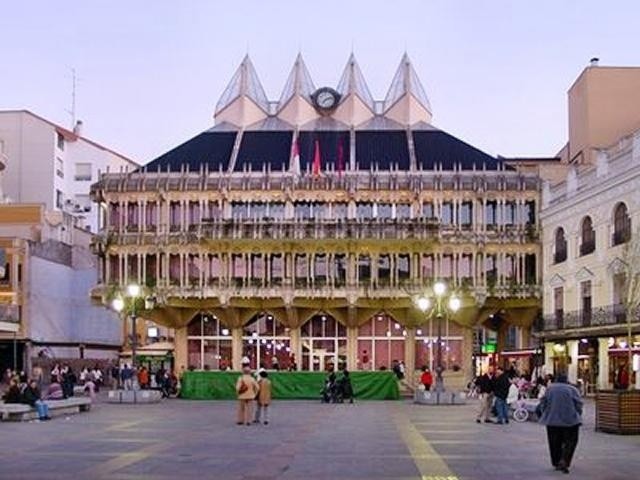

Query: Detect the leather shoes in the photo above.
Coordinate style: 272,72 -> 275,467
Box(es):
236,420 -> 269,425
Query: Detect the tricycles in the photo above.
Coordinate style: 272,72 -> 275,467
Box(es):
510,379 -> 541,421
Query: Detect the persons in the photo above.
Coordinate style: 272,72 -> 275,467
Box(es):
534,370 -> 587,474
0,347 -> 639,426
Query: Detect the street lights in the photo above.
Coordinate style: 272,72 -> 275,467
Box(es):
114,280 -> 158,392
418,280 -> 460,392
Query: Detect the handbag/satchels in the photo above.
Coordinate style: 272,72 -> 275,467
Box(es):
239,380 -> 248,394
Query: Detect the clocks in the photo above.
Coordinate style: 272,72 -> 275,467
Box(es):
311,88 -> 340,116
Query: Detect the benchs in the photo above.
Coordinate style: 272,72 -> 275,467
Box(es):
509,399 -> 541,422
0,396 -> 93,420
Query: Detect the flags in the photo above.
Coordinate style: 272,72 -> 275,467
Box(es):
286,131 -> 301,185
312,140 -> 322,179
337,134 -> 343,180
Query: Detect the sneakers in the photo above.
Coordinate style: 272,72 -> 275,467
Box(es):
475,419 -> 509,424
559,459 -> 569,473
